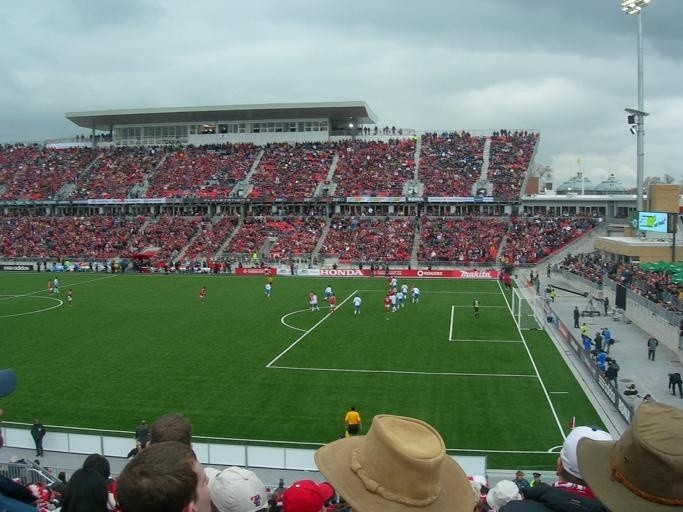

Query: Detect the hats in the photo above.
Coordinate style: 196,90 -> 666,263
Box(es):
281,479 -> 334,512
486,479 -> 548,511
559,425 -> 612,484
533,473 -> 541,477
0,368 -> 17,400
468,475 -> 486,505
314,414 -> 475,512
577,401 -> 683,512
201,465 -> 268,512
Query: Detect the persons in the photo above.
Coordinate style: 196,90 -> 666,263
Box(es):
351,295 -> 361,315
48,280 -> 55,296
54,277 -> 60,294
308,291 -> 314,309
530,269 -> 556,301
199,286 -> 207,304
503,272 -> 512,290
559,251 -> 682,368
67,289 -> 74,307
264,276 -> 273,298
471,297 -> 481,318
310,293 -> 320,311
384,276 -> 420,314
324,284 -> 332,300
330,293 -> 336,317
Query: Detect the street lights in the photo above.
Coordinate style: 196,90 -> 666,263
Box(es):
622,0 -> 652,214
